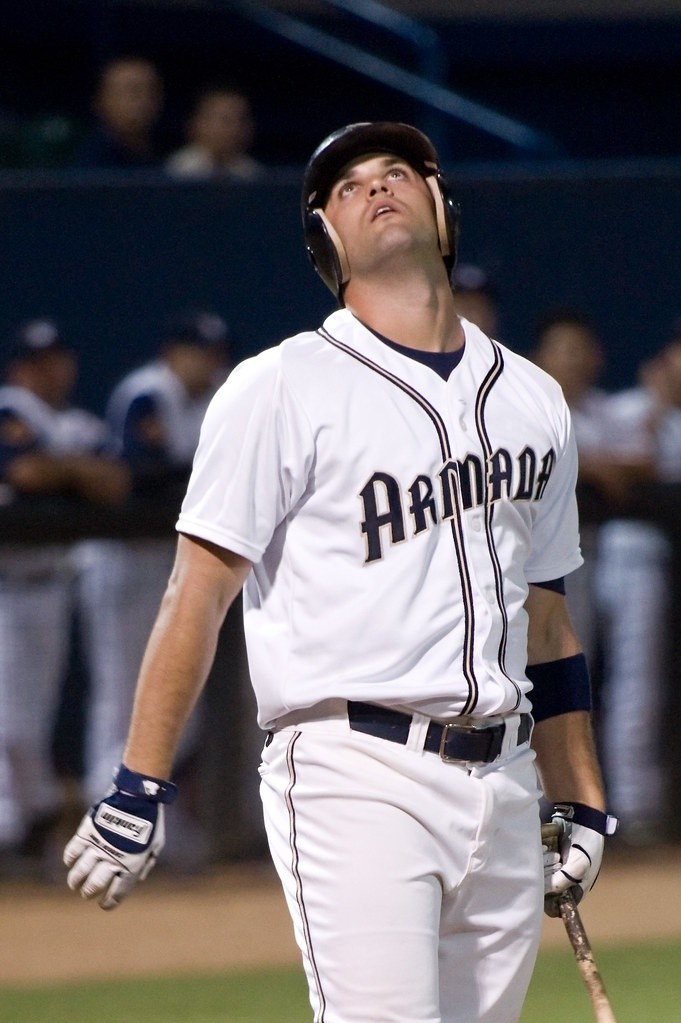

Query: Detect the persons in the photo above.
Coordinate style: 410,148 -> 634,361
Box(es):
59,120 -> 622,1023
1,62 -> 681,860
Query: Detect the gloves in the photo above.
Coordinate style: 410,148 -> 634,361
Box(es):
541,801 -> 620,918
62,763 -> 178,911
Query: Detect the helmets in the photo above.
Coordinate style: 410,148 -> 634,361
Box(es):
300,120 -> 463,306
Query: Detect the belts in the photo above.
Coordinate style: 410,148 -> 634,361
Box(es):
346,699 -> 533,764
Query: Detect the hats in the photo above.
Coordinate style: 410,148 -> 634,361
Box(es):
164,312 -> 230,351
8,318 -> 74,358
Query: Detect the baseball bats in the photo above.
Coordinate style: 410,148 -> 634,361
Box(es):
539,820 -> 618,1023
